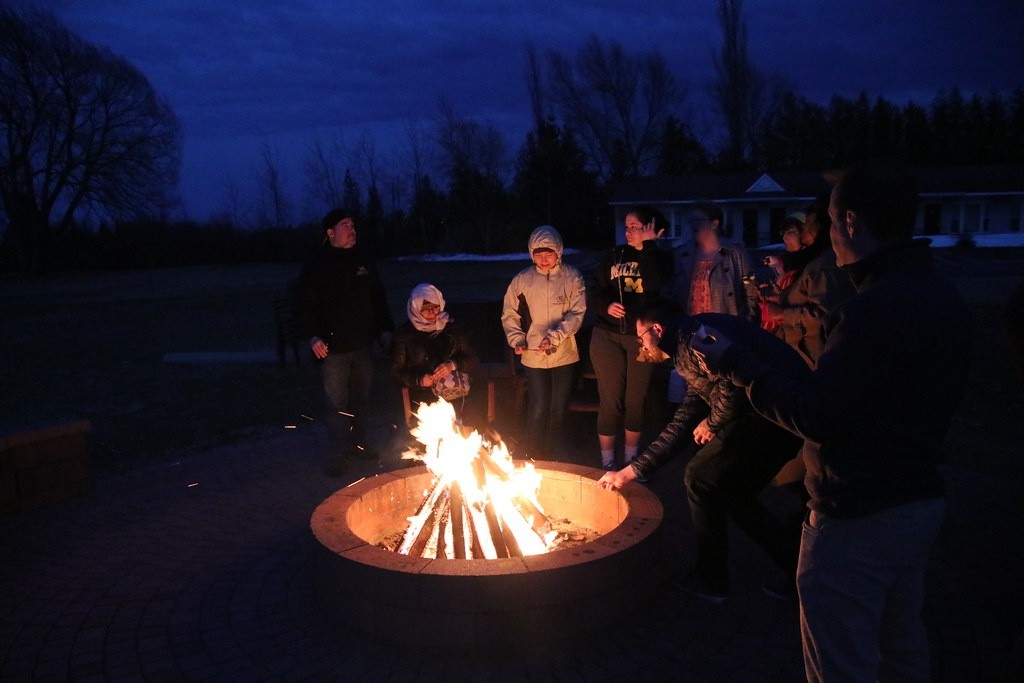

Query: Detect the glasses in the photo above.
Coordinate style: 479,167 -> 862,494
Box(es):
635,326 -> 655,346
420,305 -> 440,318
688,218 -> 711,225
780,231 -> 799,236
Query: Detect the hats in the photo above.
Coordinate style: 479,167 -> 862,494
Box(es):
323,207 -> 358,226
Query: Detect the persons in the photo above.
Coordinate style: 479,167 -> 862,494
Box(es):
390,283 -> 480,425
587,205 -> 688,472
290,207 -> 397,476
597,158 -> 1024,683
501,224 -> 587,461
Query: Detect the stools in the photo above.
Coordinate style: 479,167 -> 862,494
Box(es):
400,360 -> 514,431
0,408 -> 90,507
508,339 -> 600,434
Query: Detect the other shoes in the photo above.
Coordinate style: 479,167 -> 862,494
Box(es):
678,571 -> 734,605
294,357 -> 302,363
363,443 -> 382,462
761,536 -> 799,601
601,456 -> 649,483
326,456 -> 345,477
275,360 -> 286,367
